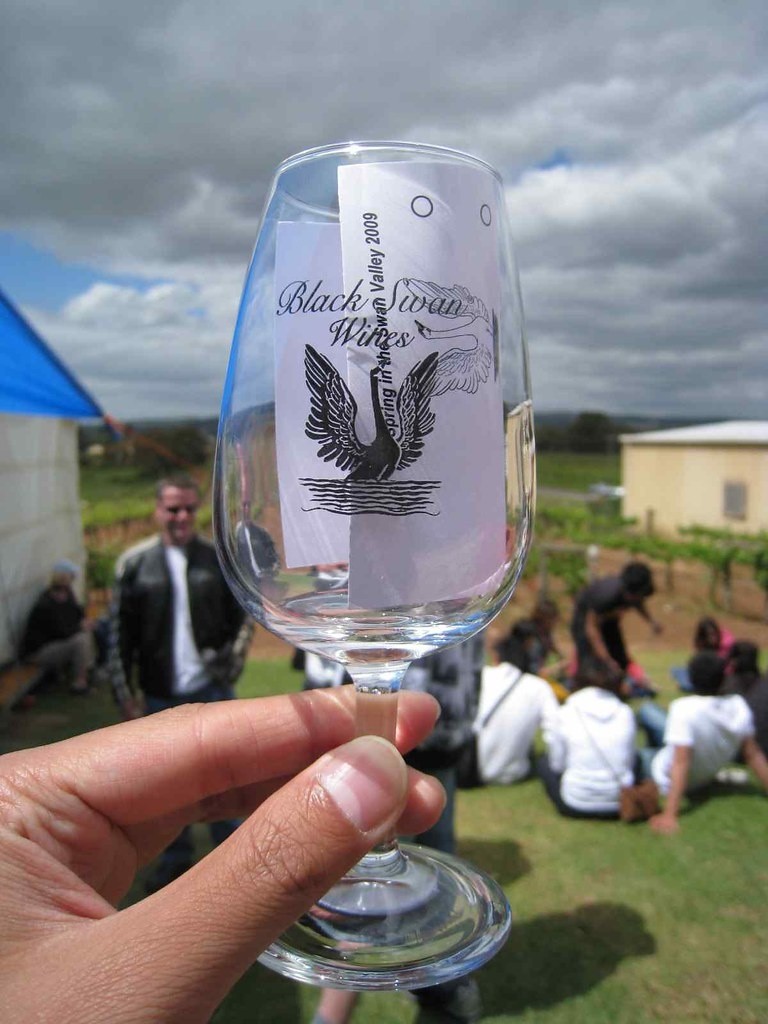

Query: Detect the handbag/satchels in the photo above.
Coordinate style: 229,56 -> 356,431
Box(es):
621,776 -> 658,824
454,735 -> 478,788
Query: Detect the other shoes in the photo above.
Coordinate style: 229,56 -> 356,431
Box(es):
67,682 -> 89,695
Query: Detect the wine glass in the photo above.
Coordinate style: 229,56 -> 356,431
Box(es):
209,130 -> 540,993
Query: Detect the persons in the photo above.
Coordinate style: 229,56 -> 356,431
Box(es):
300,627 -> 481,1024
18,558 -> 100,699
568,560 -> 663,701
472,599 -> 768,831
101,476 -> 257,895
229,440 -> 280,581
0,683 -> 448,1024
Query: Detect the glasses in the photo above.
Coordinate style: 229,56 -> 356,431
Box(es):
51,584 -> 71,591
161,503 -> 195,514
